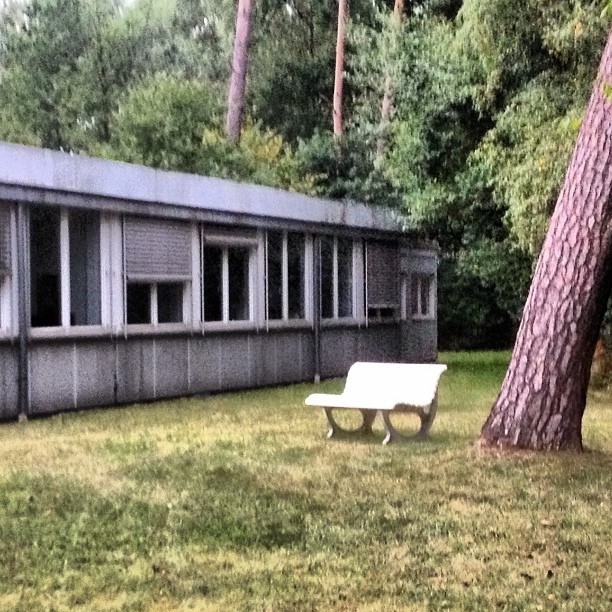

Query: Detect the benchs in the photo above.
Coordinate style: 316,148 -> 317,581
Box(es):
305,362 -> 449,445
